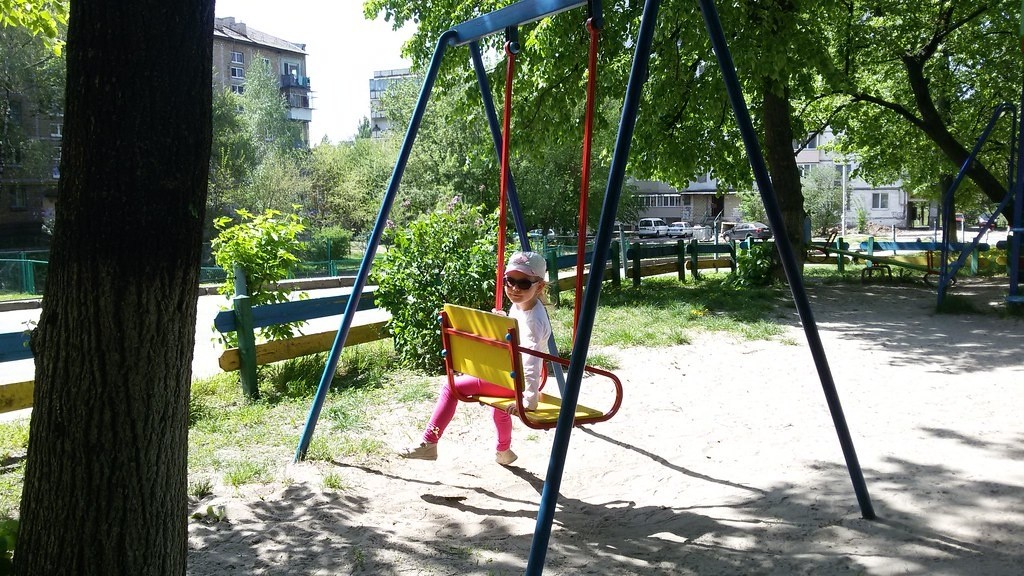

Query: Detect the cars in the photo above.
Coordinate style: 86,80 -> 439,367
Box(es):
527,228 -> 555,240
638,218 -> 669,238
955,213 -> 965,229
668,221 -> 693,238
721,220 -> 772,242
978,212 -> 997,230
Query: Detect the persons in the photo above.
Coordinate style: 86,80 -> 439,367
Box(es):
397,252 -> 552,465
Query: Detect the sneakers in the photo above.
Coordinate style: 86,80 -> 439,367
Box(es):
495,448 -> 518,465
399,439 -> 438,460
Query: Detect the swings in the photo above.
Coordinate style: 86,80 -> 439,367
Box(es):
435,17 -> 625,431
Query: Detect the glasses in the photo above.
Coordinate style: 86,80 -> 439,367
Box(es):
503,276 -> 538,290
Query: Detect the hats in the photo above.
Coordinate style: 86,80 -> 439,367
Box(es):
504,251 -> 546,279
